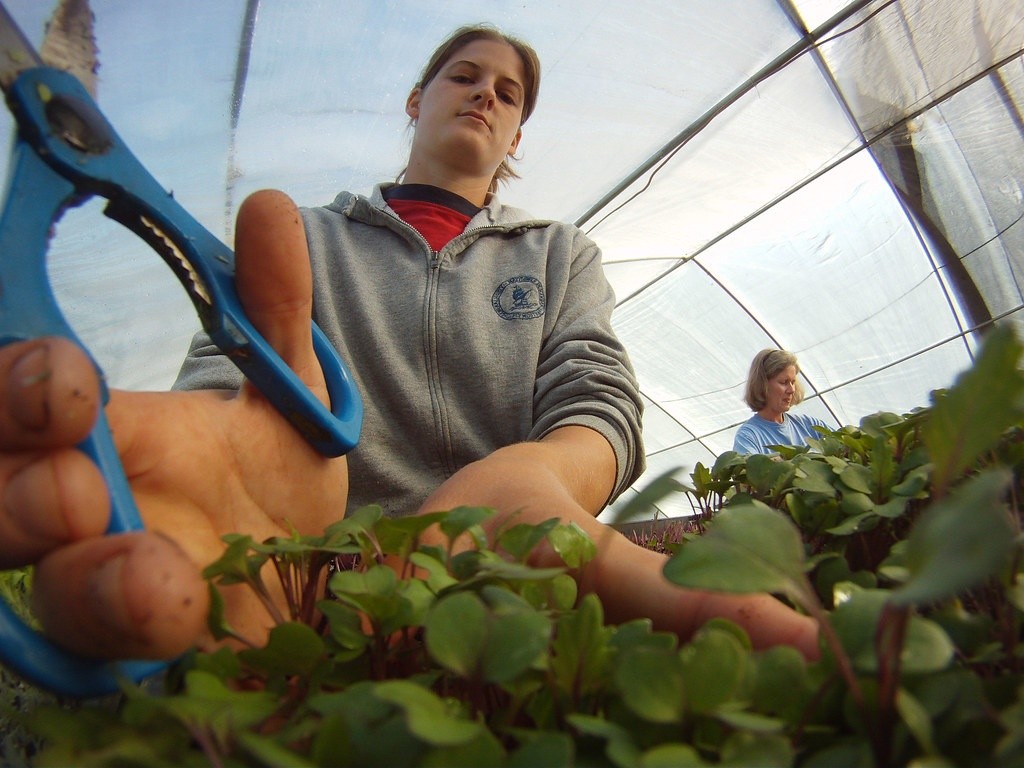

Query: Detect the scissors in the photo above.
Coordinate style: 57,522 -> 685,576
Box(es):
0,0 -> 370,700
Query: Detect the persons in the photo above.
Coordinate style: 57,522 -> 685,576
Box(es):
733,350 -> 836,460
0,22 -> 820,664
